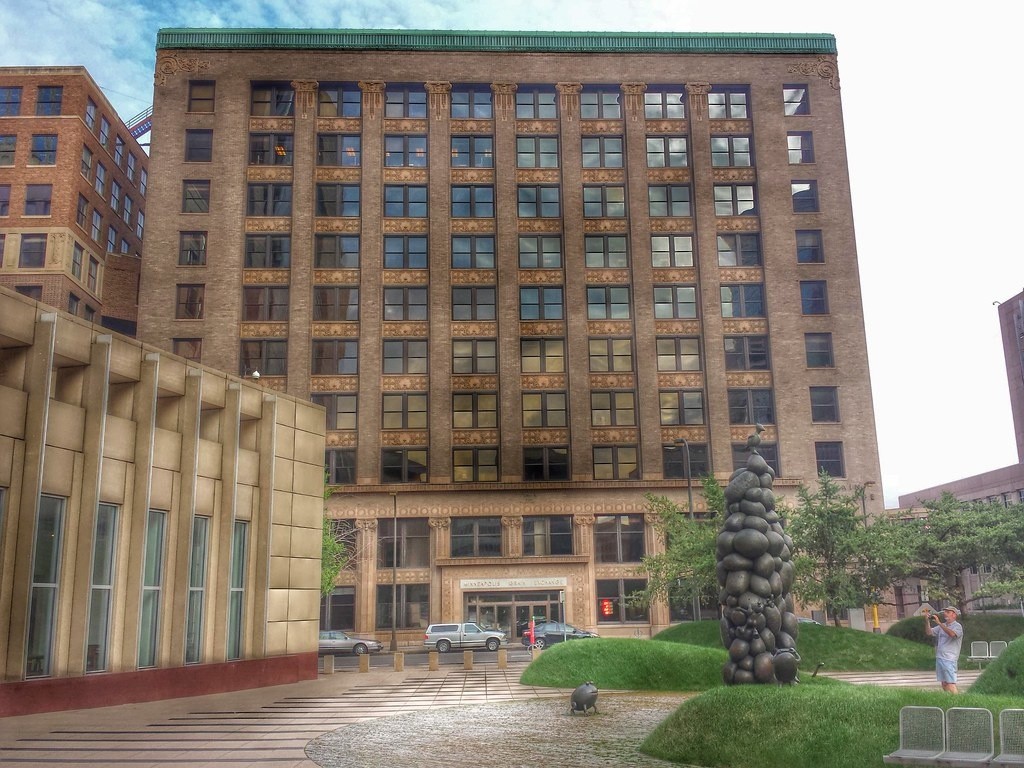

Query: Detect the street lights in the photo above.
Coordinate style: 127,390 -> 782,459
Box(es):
673,437 -> 702,623
387,489 -> 398,653
862,480 -> 877,535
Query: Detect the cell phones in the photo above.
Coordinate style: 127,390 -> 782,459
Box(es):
929,616 -> 935,619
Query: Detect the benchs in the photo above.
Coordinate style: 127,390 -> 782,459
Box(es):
883,705 -> 1024,768
967,641 -> 1013,670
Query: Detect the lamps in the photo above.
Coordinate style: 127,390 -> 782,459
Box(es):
243,365 -> 260,380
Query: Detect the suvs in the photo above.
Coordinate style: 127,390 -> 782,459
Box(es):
423,622 -> 508,653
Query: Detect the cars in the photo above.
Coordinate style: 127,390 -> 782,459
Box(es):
521,621 -> 600,650
318,630 -> 384,656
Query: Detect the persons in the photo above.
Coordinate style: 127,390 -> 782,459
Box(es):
925,606 -> 963,694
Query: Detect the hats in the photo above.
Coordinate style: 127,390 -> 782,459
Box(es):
942,606 -> 958,615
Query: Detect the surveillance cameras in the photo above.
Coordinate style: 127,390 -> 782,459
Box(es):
252,372 -> 260,380
